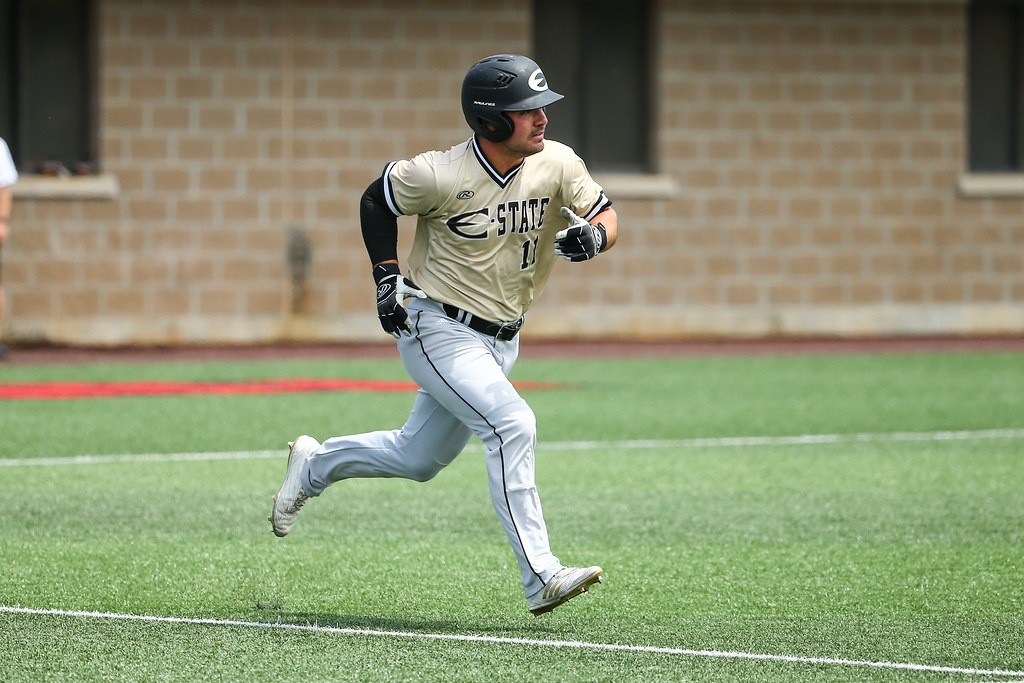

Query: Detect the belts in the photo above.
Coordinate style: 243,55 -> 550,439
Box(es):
441,303 -> 525,341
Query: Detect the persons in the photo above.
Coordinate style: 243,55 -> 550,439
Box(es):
268,54 -> 618,617
0,137 -> 18,357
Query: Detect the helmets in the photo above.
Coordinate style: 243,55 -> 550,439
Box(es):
461,54 -> 565,143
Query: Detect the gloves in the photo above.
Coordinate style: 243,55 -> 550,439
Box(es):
553,207 -> 607,263
372,263 -> 428,340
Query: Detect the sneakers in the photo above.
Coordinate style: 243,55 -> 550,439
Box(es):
268,435 -> 322,537
526,565 -> 603,618
